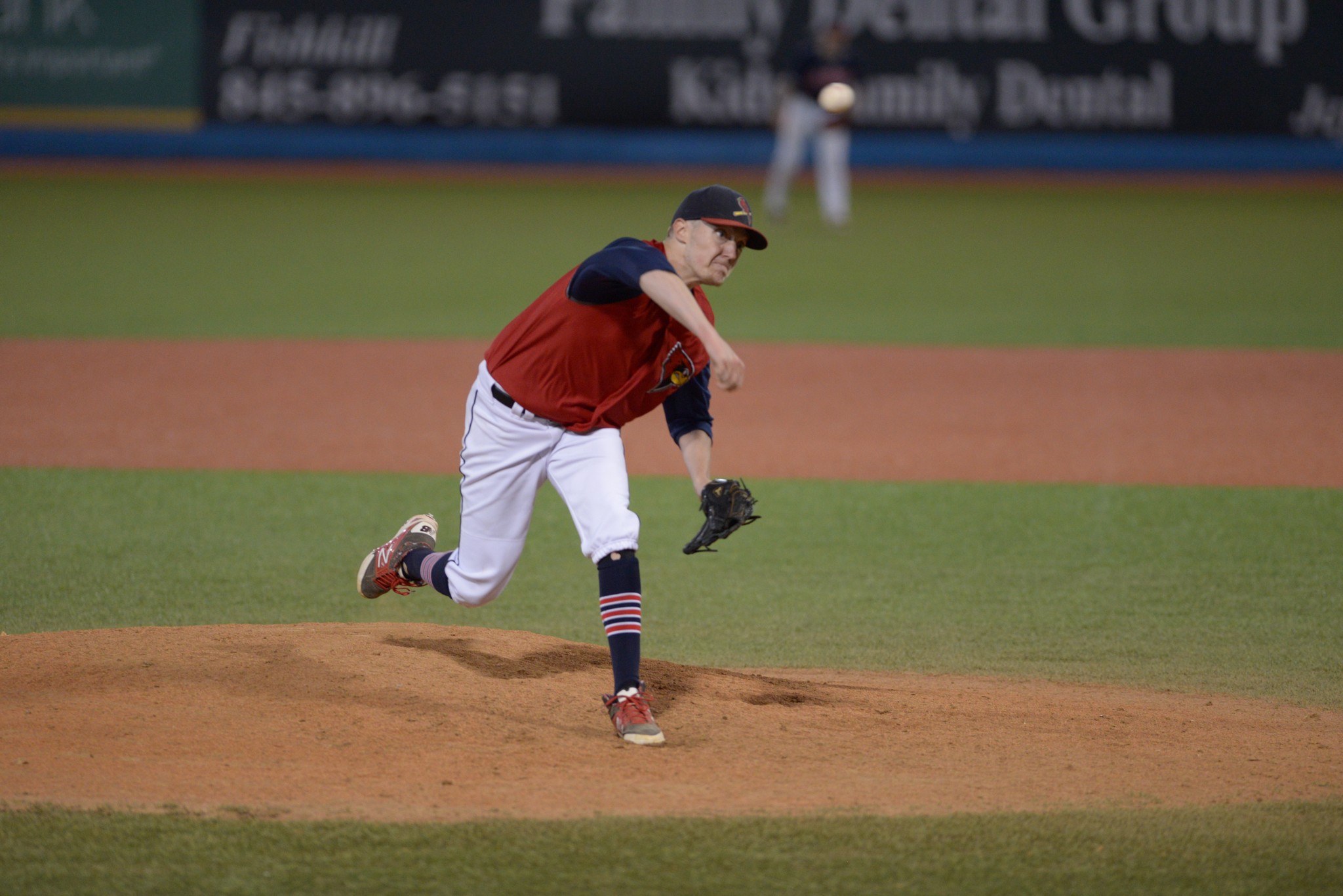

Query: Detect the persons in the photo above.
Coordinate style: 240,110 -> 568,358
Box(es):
759,0 -> 855,226
358,183 -> 767,749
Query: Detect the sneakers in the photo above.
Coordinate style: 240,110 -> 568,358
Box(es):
603,680 -> 665,745
357,515 -> 438,600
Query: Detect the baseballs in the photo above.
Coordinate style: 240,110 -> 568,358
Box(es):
817,82 -> 856,119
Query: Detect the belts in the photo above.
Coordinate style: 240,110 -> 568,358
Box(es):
492,384 -> 542,417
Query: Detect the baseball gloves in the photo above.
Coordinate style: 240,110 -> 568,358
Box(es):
681,476 -> 754,555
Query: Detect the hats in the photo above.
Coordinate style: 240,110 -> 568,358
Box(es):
672,185 -> 768,250
